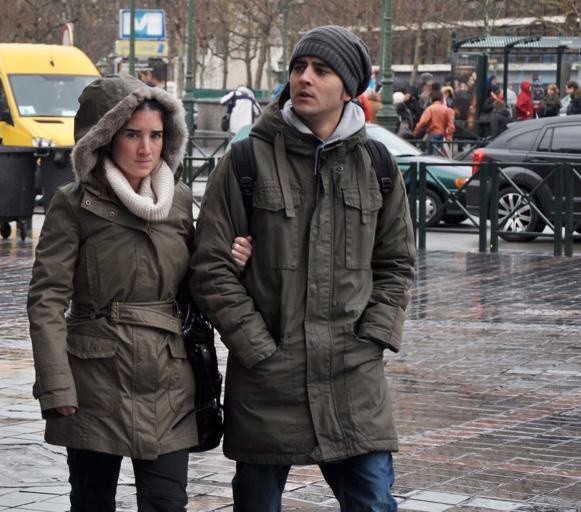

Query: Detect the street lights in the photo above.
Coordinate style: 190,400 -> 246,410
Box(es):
555,44 -> 574,97
453,33 -> 486,88
501,36 -> 540,104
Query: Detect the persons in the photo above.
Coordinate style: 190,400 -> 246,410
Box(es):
138,56 -> 168,90
220,84 -> 264,137
25,69 -> 256,510
184,23 -> 421,511
357,66 -> 581,155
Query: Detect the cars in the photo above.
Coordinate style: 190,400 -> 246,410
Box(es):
364,121 -> 478,229
467,113 -> 581,240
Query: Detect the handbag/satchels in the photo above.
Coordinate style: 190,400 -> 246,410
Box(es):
222,116 -> 229,131
182,305 -> 224,452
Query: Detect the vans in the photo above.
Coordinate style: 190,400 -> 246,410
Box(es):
0,42 -> 105,214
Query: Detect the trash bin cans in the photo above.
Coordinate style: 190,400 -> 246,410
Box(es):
0,145 -> 76,240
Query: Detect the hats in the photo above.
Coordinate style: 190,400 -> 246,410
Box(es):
289,25 -> 371,99
